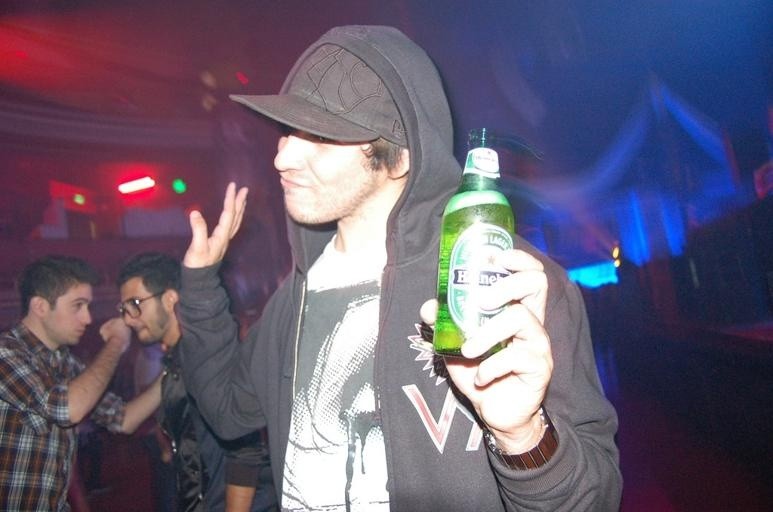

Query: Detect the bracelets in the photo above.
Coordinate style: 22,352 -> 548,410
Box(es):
480,407 -> 558,470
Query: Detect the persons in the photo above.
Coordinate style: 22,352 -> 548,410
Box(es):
172,19 -> 627,511
113,250 -> 280,511
0,251 -> 167,511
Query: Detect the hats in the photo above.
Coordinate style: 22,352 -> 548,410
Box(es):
225,40 -> 409,149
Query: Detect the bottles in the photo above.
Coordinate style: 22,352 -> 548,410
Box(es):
430,125 -> 515,361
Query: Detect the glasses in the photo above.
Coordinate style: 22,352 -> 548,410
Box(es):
114,289 -> 163,319
